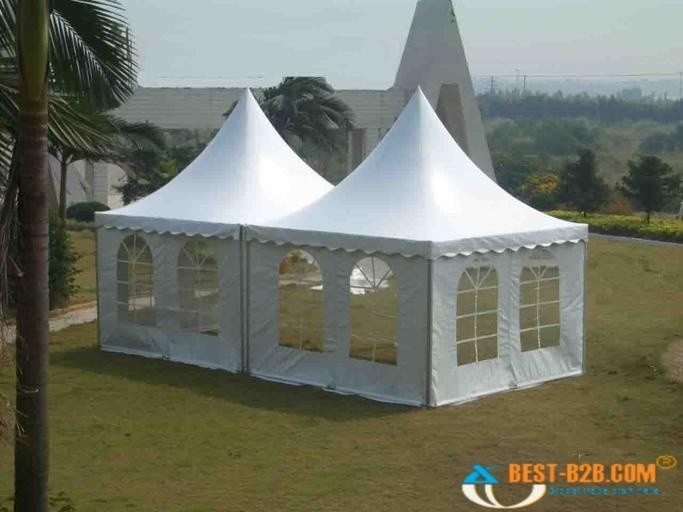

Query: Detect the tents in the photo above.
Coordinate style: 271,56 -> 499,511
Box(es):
94,86 -> 337,375
246,86 -> 589,409
